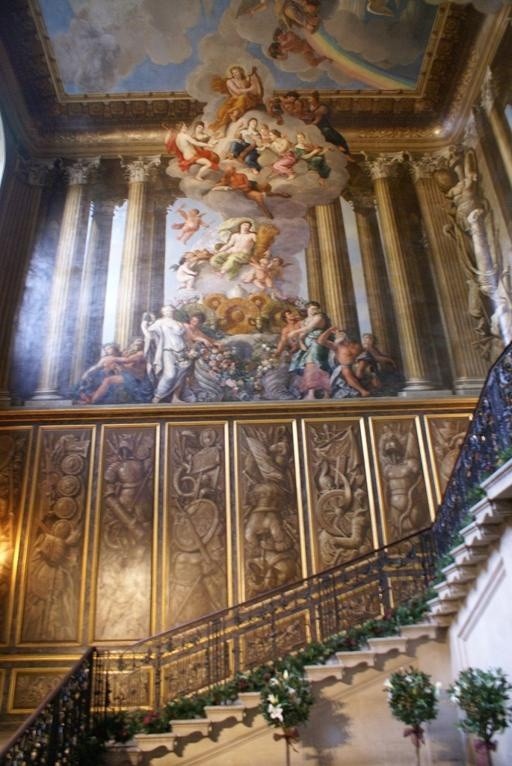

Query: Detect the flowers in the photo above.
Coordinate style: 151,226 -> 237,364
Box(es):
416,445 -> 511,600
77,597 -> 512,766
